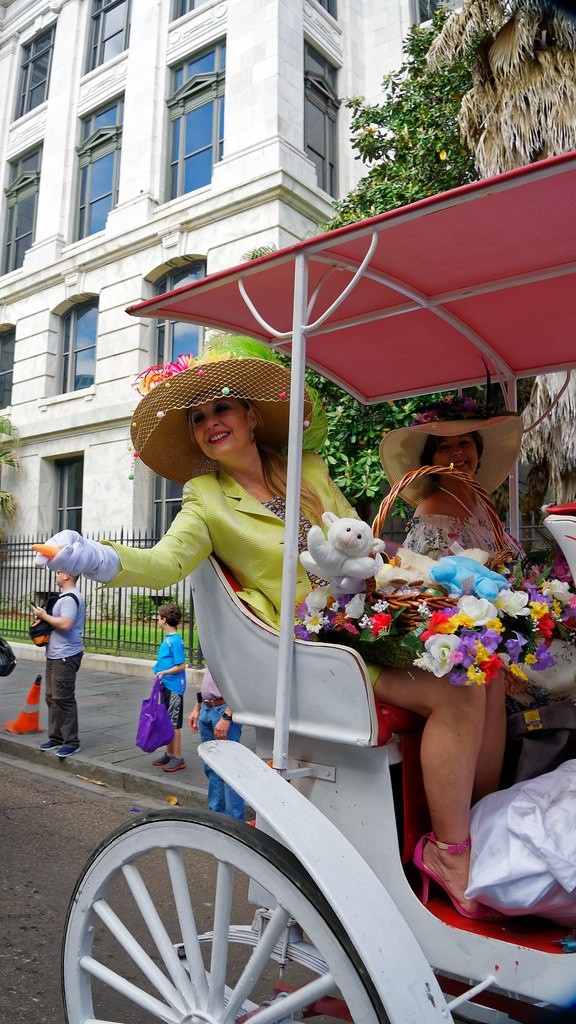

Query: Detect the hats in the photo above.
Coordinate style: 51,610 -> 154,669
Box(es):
379,397 -> 523,507
129,336 -> 314,486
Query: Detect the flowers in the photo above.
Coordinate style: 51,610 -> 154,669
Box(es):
410,394 -> 485,424
291,549 -> 575,689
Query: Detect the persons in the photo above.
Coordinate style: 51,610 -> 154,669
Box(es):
188,666 -> 245,822
32,572 -> 86,757
34,335 -> 509,923
152,603 -> 186,772
379,395 -> 576,790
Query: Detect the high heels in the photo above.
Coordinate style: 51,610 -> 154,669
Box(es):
412,832 -> 505,922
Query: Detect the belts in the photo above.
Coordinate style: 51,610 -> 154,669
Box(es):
202,699 -> 226,707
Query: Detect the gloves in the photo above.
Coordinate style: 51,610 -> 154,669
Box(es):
35,528 -> 106,579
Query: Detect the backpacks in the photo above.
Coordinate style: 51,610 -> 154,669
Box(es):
0,637 -> 17,677
29,593 -> 79,647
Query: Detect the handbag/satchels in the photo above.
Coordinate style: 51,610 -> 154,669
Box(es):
135,677 -> 174,753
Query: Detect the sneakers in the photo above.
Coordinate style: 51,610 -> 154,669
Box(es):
39,739 -> 63,751
151,751 -> 172,766
162,755 -> 186,772
55,744 -> 82,757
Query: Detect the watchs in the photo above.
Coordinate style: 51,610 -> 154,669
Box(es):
222,712 -> 232,721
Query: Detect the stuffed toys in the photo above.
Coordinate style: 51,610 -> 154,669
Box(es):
299,512 -> 385,597
376,548 -> 511,602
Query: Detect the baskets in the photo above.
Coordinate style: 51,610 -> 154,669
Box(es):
360,463 -> 515,638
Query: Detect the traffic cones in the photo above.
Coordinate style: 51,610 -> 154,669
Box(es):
2,675 -> 47,736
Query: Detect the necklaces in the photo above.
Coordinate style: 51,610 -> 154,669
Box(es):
439,487 -> 504,550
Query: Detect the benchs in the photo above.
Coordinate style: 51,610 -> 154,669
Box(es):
191,534 -> 423,875
